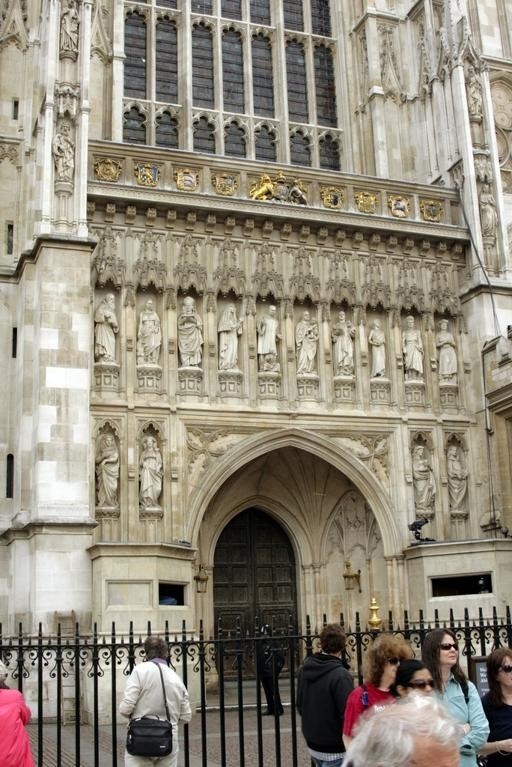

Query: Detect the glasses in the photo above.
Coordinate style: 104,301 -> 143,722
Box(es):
408,680 -> 434,688
499,666 -> 512,672
388,657 -> 403,664
439,643 -> 458,650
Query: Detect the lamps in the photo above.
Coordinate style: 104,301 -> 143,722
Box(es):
194,562 -> 209,595
342,560 -> 364,597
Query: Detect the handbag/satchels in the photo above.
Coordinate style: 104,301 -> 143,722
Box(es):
126,713 -> 172,757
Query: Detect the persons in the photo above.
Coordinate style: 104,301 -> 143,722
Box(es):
257,623 -> 286,714
345,688 -> 461,766
403,315 -> 423,379
140,435 -> 163,507
96,434 -> 119,507
0,660 -> 37,766
219,303 -> 244,369
256,304 -> 282,370
295,311 -> 318,373
412,444 -> 436,509
421,629 -> 491,766
368,319 -> 386,376
178,297 -> 202,367
94,292 -> 117,361
332,310 -> 356,375
475,647 -> 511,766
436,318 -> 456,381
342,634 -> 413,753
119,636 -> 192,766
136,299 -> 161,366
296,623 -> 354,766
447,445 -> 468,508
